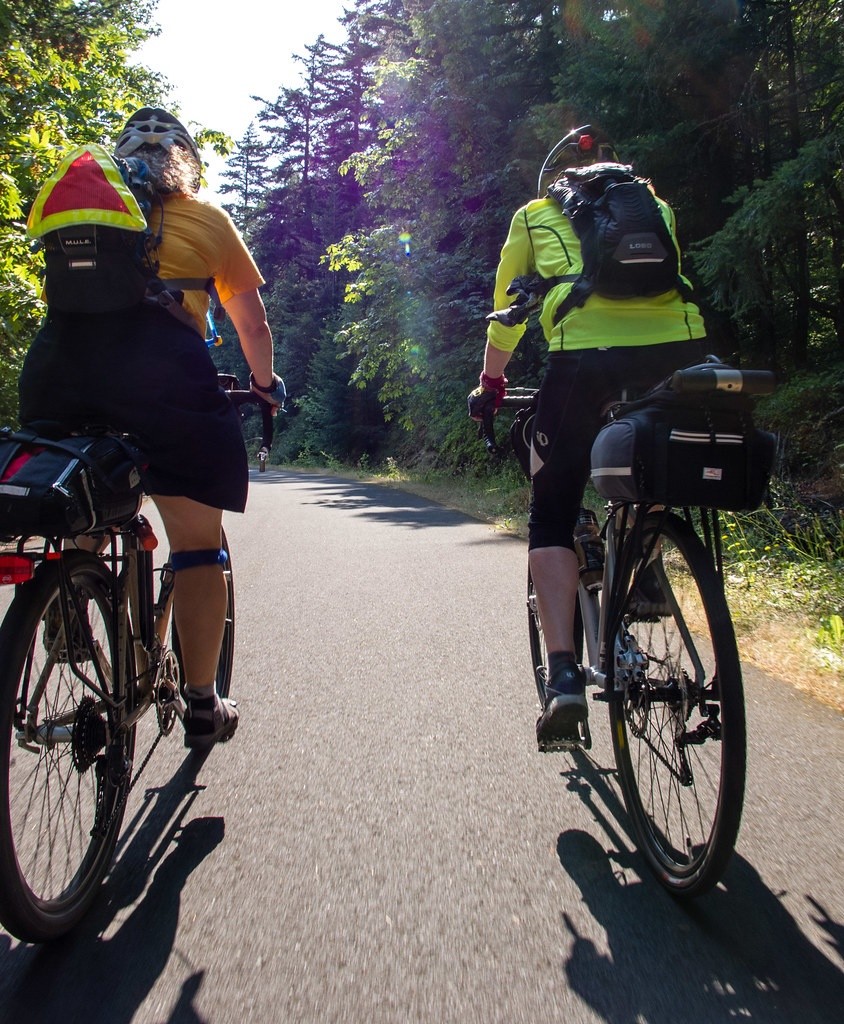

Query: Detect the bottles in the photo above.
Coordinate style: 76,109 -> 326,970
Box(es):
573,510 -> 605,591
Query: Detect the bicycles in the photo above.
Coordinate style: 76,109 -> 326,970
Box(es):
0,372 -> 275,946
479,385 -> 747,901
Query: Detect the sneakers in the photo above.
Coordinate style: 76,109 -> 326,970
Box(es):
622,567 -> 675,615
183,704 -> 239,748
45,596 -> 91,658
536,672 -> 588,732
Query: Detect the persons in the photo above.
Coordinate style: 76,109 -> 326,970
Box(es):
16,108 -> 287,752
467,127 -> 708,737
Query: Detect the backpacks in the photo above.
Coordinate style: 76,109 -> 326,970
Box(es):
556,171 -> 672,298
22,149 -> 153,374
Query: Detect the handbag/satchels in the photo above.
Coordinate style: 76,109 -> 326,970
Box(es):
1,419 -> 145,545
588,392 -> 778,510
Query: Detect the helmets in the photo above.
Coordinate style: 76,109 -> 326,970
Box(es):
114,108 -> 201,166
536,126 -> 620,199
